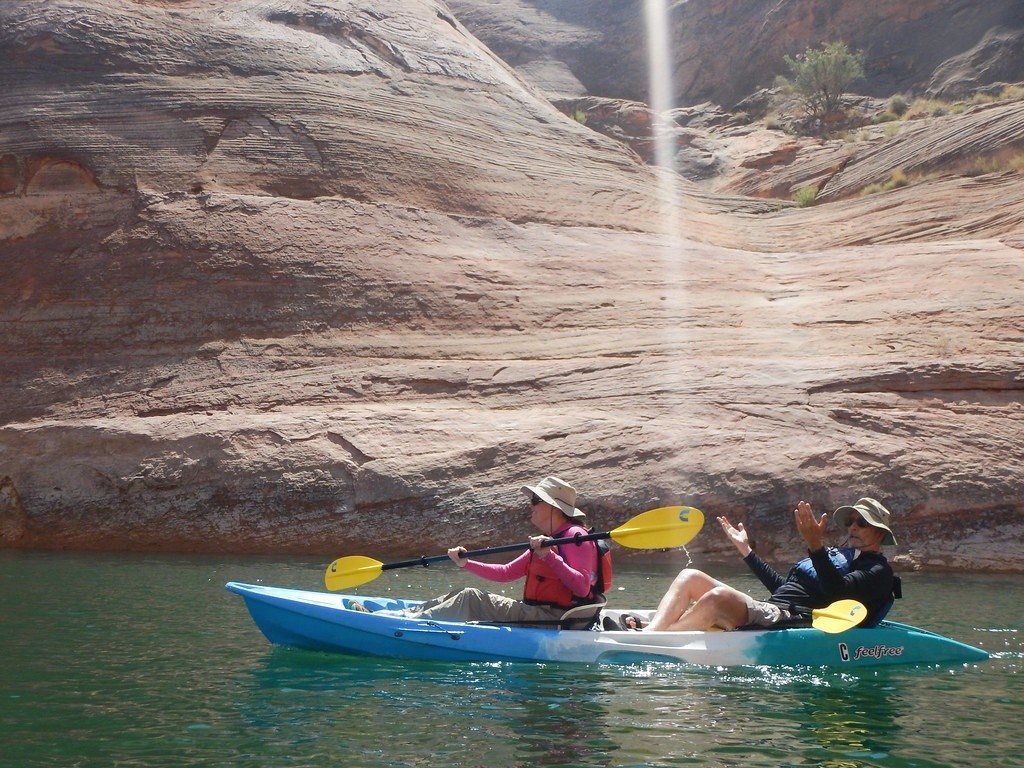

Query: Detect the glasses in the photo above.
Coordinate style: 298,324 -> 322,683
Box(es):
844,515 -> 868,528
530,496 -> 544,506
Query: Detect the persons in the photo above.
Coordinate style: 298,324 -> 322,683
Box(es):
602,496 -> 896,629
347,476 -> 598,632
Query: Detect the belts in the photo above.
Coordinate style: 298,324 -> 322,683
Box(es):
526,599 -> 566,610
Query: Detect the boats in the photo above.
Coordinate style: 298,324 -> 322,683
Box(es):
222,574 -> 998,674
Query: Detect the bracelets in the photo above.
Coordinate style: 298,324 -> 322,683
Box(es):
540,552 -> 548,560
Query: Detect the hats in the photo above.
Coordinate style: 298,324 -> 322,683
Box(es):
833,497 -> 893,545
520,474 -> 587,519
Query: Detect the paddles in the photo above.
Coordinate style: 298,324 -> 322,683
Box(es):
325,502 -> 705,591
761,598 -> 867,636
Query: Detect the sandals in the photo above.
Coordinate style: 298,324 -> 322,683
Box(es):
620,614 -> 642,630
603,617 -> 623,631
